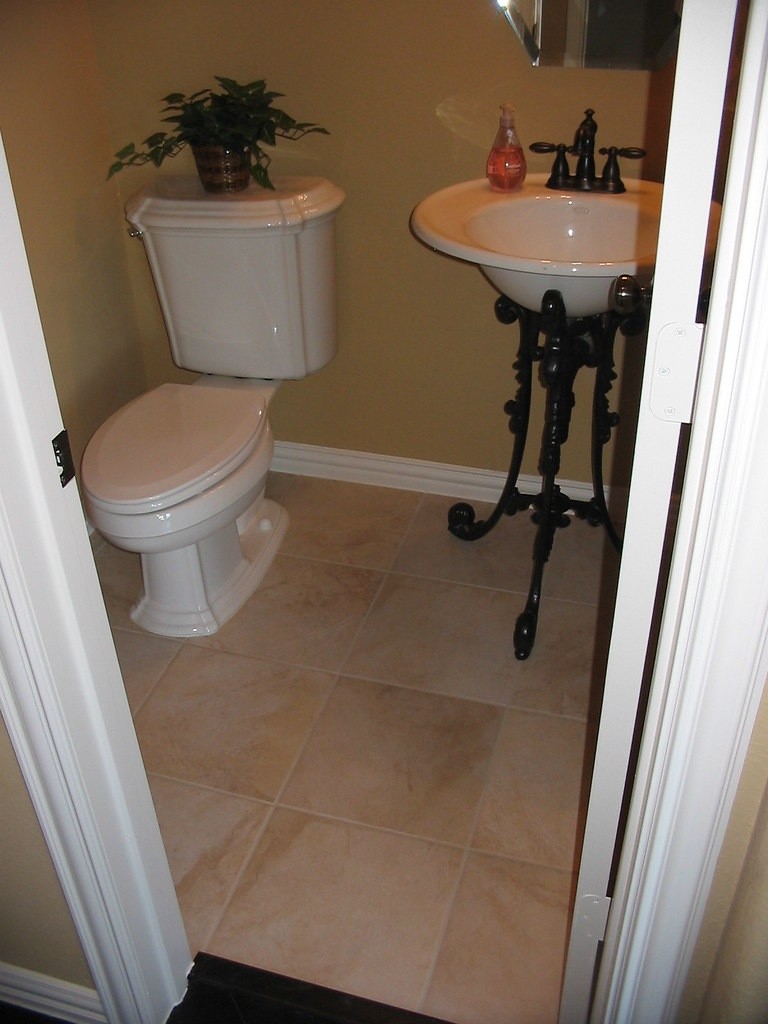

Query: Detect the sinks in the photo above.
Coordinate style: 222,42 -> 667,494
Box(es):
409,172 -> 722,316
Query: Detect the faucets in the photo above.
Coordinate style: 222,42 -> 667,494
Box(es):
526,109 -> 648,194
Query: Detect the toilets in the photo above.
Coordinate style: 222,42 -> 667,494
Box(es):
80,175 -> 347,639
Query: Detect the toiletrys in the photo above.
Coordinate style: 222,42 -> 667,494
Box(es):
485,102 -> 527,193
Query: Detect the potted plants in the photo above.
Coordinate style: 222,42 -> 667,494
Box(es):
102,75 -> 332,190
